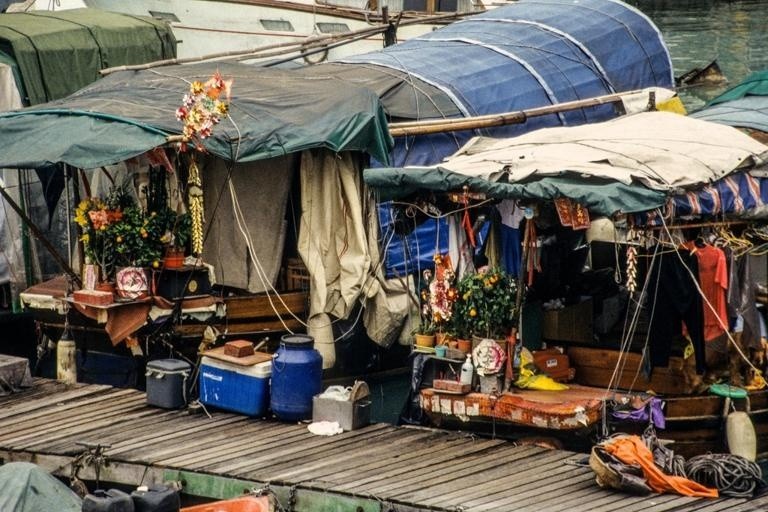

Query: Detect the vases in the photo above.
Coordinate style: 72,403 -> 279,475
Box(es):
164,257 -> 184,269
457,340 -> 470,354
416,333 -> 435,349
96,282 -> 115,292
471,334 -> 508,360
437,334 -> 456,347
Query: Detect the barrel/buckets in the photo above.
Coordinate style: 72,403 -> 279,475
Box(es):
269,334 -> 324,422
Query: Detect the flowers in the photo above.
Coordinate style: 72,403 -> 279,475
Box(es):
453,264 -> 477,337
79,192 -> 139,282
463,268 -> 523,339
433,285 -> 460,334
407,256 -> 444,334
143,205 -> 193,249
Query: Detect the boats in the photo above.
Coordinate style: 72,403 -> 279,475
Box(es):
1,2 -> 679,403
361,69 -> 768,459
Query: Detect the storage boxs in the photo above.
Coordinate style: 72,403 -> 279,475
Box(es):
146,359 -> 192,410
199,357 -> 273,417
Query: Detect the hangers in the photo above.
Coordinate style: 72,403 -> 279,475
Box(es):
634,220 -> 768,259
